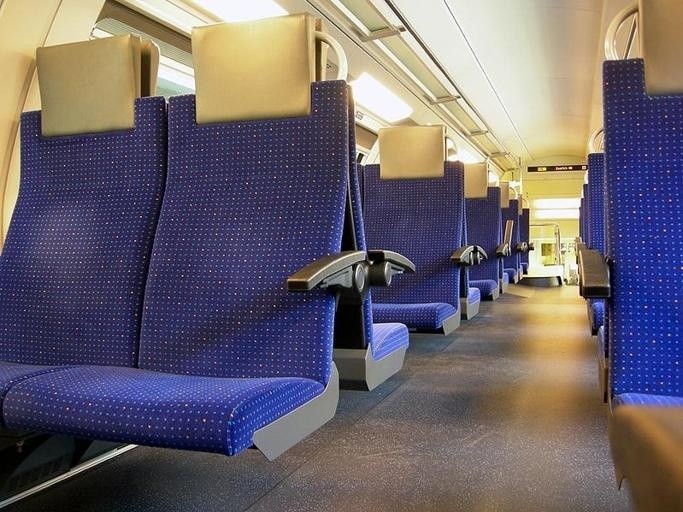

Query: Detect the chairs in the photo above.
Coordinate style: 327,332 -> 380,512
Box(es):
0,30 -> 164,510
3,11 -> 369,463
315,33 -> 416,391
501,181 -> 527,285
573,0 -> 683,510
361,122 -> 472,337
518,199 -> 534,275
459,161 -> 499,301
